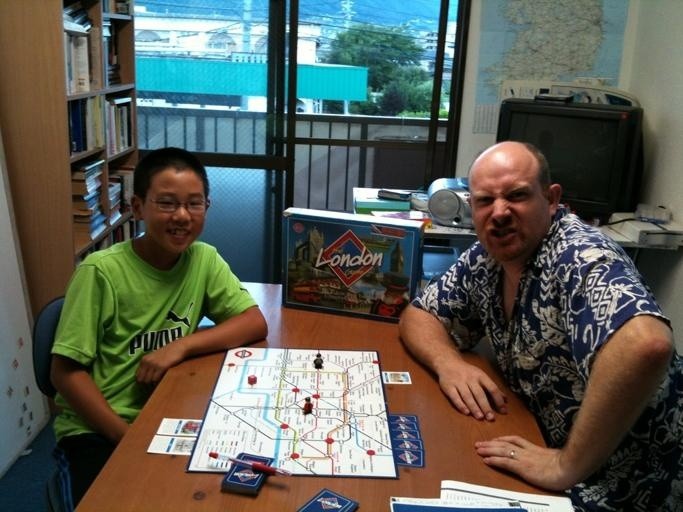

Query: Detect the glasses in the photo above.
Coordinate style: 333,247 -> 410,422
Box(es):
140,189 -> 211,216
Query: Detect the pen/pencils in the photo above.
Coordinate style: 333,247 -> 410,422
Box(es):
209,450 -> 291,478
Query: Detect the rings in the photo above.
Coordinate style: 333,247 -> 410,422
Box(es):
510,450 -> 515,458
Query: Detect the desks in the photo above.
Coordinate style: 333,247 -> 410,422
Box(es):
73,281 -> 569,511
351,186 -> 680,287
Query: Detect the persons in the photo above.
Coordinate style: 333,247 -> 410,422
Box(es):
50,146 -> 269,511
399,140 -> 683,512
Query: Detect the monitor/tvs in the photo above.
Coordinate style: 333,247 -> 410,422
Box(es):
494,95 -> 644,226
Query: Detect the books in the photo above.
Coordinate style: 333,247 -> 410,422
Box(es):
64,1 -> 140,254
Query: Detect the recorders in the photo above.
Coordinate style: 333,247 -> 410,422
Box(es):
427,175 -> 474,230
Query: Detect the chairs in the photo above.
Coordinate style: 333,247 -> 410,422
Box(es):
30,295 -> 71,512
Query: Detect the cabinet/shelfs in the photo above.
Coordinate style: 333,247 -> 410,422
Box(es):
0,0 -> 141,323
